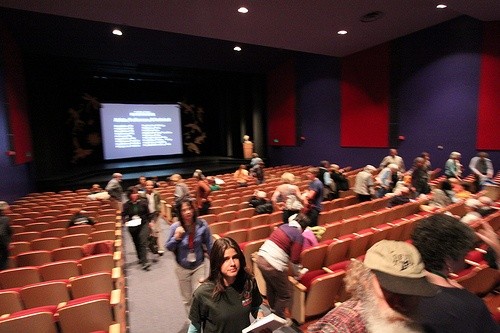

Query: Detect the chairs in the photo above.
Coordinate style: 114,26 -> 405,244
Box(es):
0,187 -> 131,333
158,164 -> 500,333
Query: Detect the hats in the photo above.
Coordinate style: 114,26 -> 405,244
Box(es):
169,173 -> 182,181
364,239 -> 441,296
112,173 -> 123,177
365,165 -> 377,171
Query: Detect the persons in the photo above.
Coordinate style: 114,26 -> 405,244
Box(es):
250,190 -> 275,217
188,238 -> 264,333
233,153 -> 263,187
306,214 -> 500,333
166,199 -> 214,325
353,165 -> 376,202
169,174 -> 190,205
318,160 -> 349,198
106,173 -> 164,263
256,213 -> 318,327
305,167 -> 323,227
0,201 -> 14,272
273,172 -> 309,224
377,148 -> 494,225
121,186 -> 153,270
86,184 -> 110,200
191,169 -> 220,215
68,212 -> 95,228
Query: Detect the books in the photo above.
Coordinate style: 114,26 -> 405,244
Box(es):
242,313 -> 287,333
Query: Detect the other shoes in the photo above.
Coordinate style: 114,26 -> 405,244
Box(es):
142,264 -> 150,270
152,253 -> 160,262
138,259 -> 142,264
